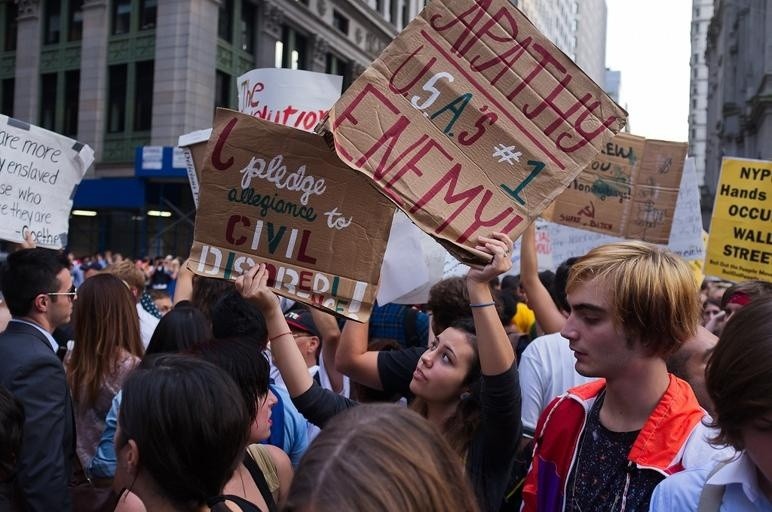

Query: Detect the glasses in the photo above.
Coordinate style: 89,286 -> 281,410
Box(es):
44,284 -> 76,301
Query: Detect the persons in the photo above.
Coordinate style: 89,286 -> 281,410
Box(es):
0,216 -> 771,510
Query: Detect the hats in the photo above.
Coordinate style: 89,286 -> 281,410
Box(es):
283,309 -> 321,340
